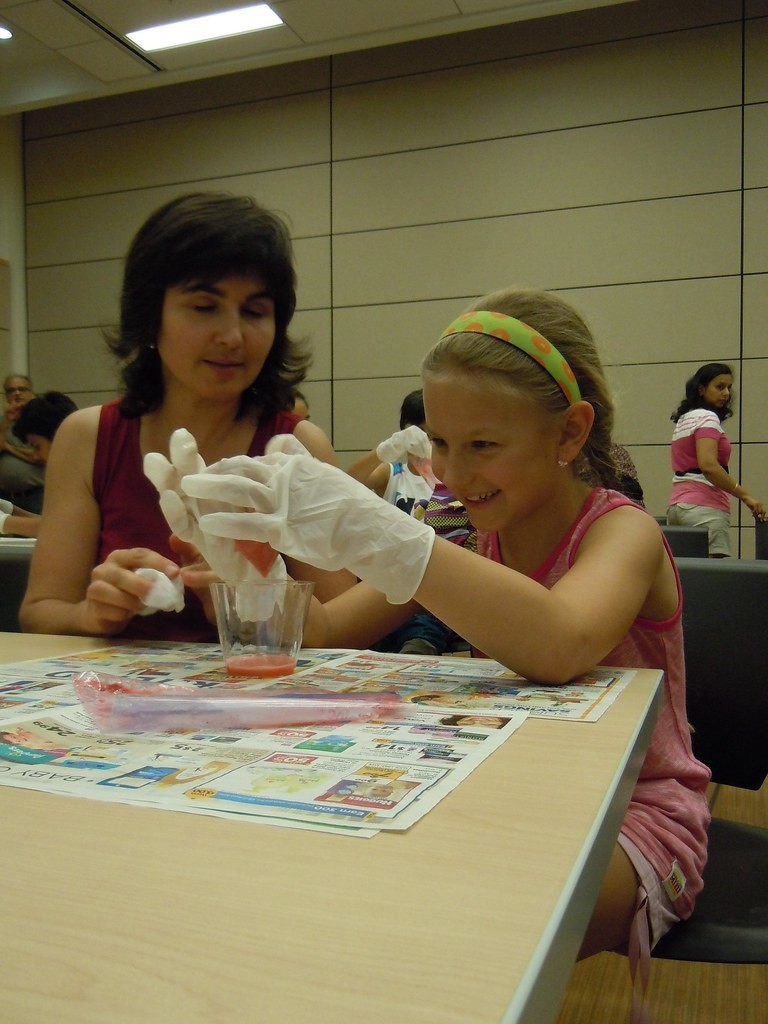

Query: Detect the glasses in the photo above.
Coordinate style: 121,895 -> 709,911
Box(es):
5,386 -> 31,394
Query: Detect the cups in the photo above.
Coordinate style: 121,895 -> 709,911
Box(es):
210,581 -> 315,676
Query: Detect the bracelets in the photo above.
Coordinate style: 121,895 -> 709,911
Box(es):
731,483 -> 737,494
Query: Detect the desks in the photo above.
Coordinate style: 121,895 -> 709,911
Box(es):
0,633 -> 665,1023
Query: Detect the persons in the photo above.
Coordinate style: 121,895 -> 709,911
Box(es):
289,389 -> 310,423
345,388 -> 475,659
0,374 -> 46,514
21,194 -> 360,649
0,391 -> 79,539
141,287 -> 716,972
662,363 -> 768,559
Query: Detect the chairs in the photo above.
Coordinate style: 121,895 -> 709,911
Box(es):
0,546 -> 35,633
648,557 -> 768,964
653,514 -> 768,559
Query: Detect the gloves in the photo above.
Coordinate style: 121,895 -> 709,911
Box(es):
376,425 -> 433,462
142,427 -> 287,622
0,498 -> 14,534
181,435 -> 435,604
